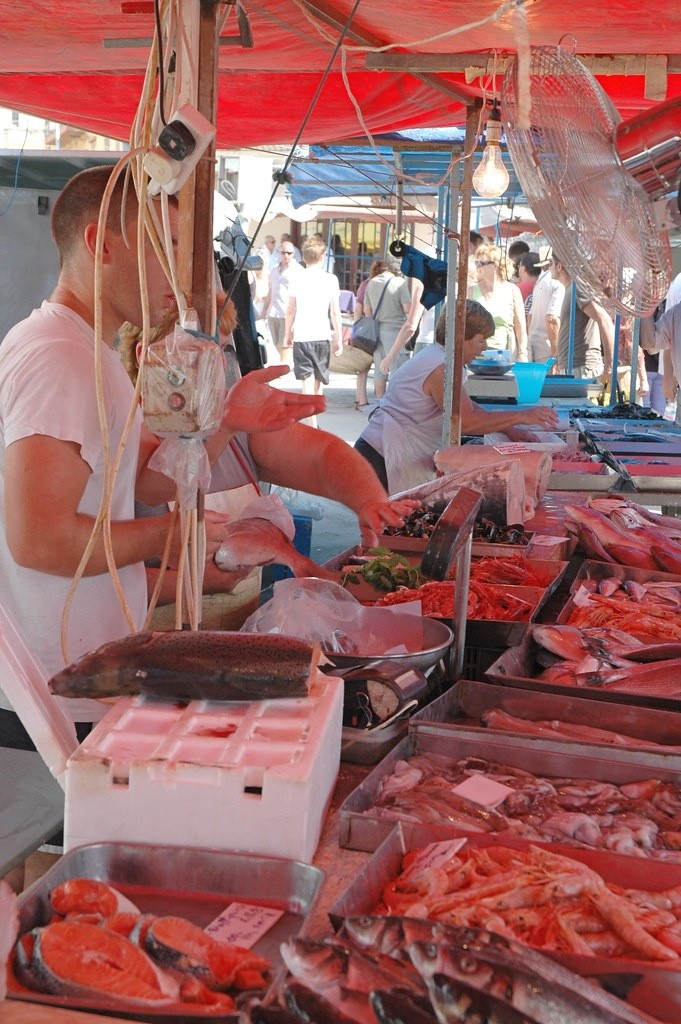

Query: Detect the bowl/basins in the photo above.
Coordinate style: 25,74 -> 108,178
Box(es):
254,607 -> 455,674
464,358 -> 516,376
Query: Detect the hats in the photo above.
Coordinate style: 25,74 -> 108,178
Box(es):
533,245 -> 553,267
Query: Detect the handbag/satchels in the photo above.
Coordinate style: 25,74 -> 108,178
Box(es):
328,345 -> 374,374
351,316 -> 380,354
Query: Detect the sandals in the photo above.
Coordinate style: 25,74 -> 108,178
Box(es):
359,402 -> 369,412
354,401 -> 360,411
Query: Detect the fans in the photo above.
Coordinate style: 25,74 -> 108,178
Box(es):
501,32 -> 681,319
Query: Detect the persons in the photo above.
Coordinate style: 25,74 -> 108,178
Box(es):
353,299 -> 560,500
236,218 -> 680,425
0,166 -> 329,755
117,277 -> 423,636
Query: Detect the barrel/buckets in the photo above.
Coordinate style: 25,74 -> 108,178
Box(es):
511,358 -> 558,404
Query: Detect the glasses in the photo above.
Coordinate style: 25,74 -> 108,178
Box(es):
475,261 -> 494,266
281,252 -> 293,255
271,241 -> 276,244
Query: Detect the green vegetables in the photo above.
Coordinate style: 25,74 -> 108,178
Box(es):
339,548 -> 430,592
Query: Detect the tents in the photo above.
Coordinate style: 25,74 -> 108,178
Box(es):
272,127 -> 640,405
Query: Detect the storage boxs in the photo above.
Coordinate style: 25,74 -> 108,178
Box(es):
61,680 -> 345,865
